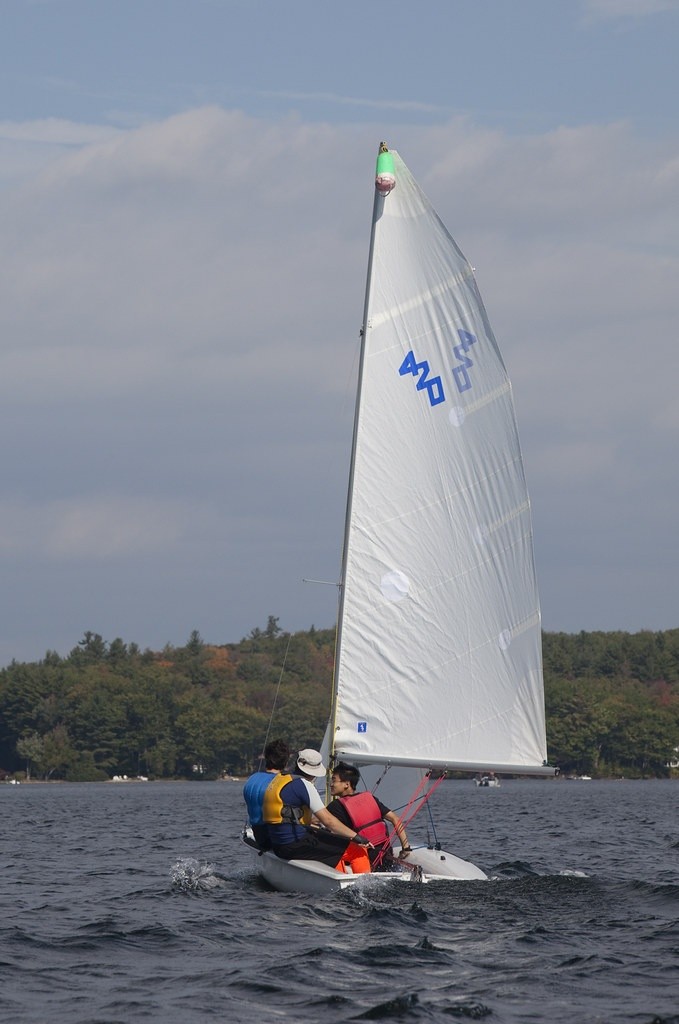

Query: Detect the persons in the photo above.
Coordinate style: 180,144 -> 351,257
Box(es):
310,762 -> 410,873
263,748 -> 376,866
242,739 -> 289,852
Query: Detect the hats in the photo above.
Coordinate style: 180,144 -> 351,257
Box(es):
297,749 -> 327,777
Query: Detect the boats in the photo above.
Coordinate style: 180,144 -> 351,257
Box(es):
476,772 -> 499,788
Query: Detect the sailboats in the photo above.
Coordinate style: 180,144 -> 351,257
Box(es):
237,138 -> 556,893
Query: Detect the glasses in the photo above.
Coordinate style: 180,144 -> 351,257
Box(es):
331,778 -> 346,783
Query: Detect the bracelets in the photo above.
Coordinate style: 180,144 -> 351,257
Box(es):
402,846 -> 412,851
